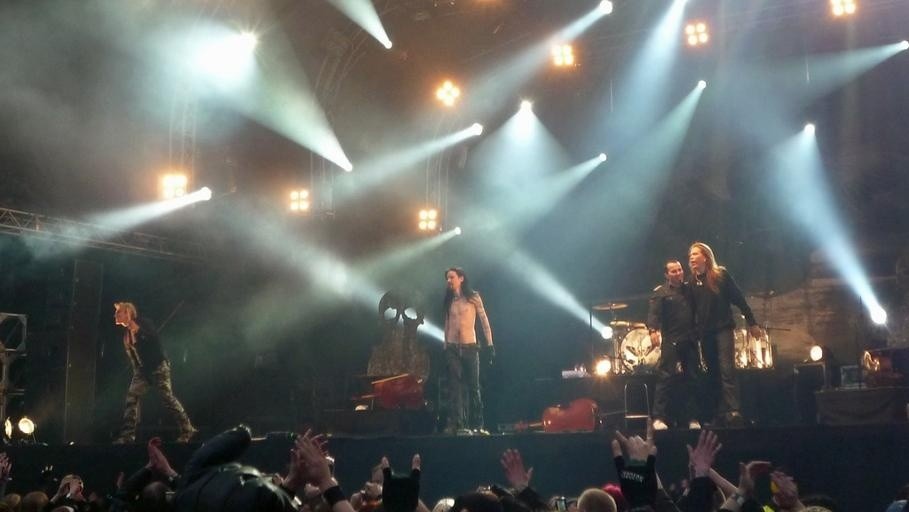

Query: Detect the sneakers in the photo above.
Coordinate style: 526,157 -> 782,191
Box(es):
176,428 -> 199,444
688,420 -> 701,430
112,435 -> 135,445
653,419 -> 669,431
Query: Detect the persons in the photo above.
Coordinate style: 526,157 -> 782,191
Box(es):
687,242 -> 763,428
644,257 -> 705,431
109,302 -> 200,445
612,416 -> 845,511
443,266 -> 496,435
0,416 -> 617,511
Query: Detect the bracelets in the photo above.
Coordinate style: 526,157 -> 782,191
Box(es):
648,328 -> 656,335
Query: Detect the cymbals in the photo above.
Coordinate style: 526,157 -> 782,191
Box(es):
592,303 -> 628,310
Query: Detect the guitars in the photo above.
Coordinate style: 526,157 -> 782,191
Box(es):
347,379 -> 425,411
515,397 -> 601,433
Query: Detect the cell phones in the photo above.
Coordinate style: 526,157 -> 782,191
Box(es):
556,499 -> 567,512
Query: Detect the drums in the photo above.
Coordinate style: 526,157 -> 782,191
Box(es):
733,327 -> 749,368
748,330 -> 773,369
620,329 -> 662,371
